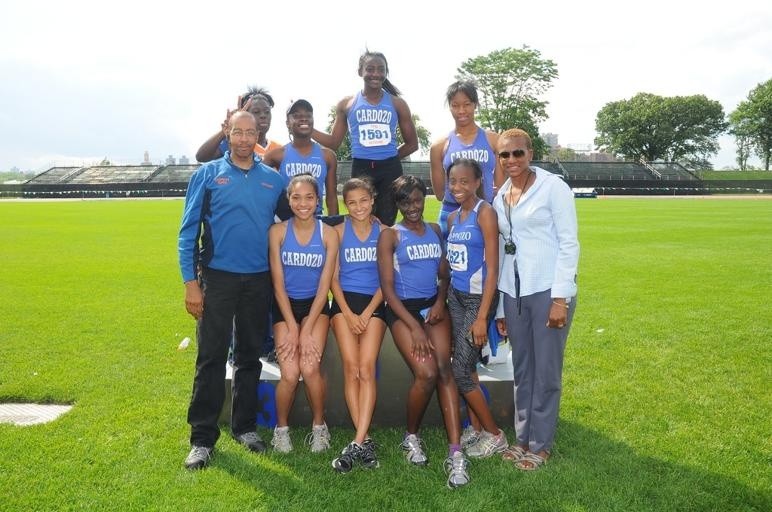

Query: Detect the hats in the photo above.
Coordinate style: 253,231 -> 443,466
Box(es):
286,99 -> 313,116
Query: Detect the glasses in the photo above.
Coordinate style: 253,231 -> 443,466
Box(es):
498,149 -> 524,159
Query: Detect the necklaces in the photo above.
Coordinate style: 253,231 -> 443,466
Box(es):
504,172 -> 531,255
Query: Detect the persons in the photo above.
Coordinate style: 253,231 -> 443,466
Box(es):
289,50 -> 419,227
177,111 -> 382,468
262,99 -> 339,217
330,178 -> 387,473
196,85 -> 285,365
377,175 -> 470,488
430,81 -> 505,238
268,172 -> 340,453
444,160 -> 509,457
491,128 -> 580,470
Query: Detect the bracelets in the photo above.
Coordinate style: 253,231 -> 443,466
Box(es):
553,300 -> 569,309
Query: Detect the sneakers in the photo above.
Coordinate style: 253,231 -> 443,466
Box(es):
442,450 -> 471,490
184,444 -> 215,471
331,441 -> 363,473
359,438 -> 376,467
397,429 -> 429,466
270,424 -> 294,454
459,423 -> 485,449
304,417 -> 331,453
229,430 -> 267,453
465,427 -> 509,459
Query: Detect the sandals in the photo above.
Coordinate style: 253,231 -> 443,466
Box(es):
502,445 -> 530,461
514,449 -> 550,470
